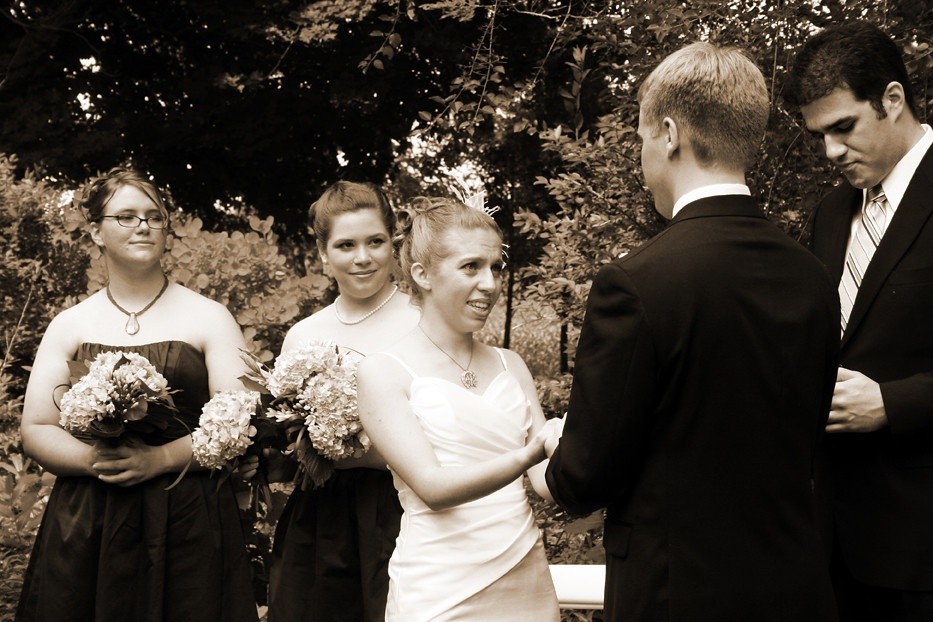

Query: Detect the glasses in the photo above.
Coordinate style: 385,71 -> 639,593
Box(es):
96,213 -> 167,229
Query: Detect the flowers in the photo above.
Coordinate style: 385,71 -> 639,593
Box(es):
192,341 -> 371,517
53,351 -> 194,492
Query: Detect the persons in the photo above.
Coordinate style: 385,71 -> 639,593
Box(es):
544,43 -> 841,622
357,198 -> 562,622
15,167 -> 262,622
267,180 -> 422,622
782,21 -> 933,622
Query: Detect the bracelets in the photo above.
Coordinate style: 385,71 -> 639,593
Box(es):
162,445 -> 172,471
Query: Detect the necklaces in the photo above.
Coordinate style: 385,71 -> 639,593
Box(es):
417,322 -> 479,389
334,284 -> 398,325
107,274 -> 169,335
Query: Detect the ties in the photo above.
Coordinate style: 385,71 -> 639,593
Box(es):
838,183 -> 888,342
